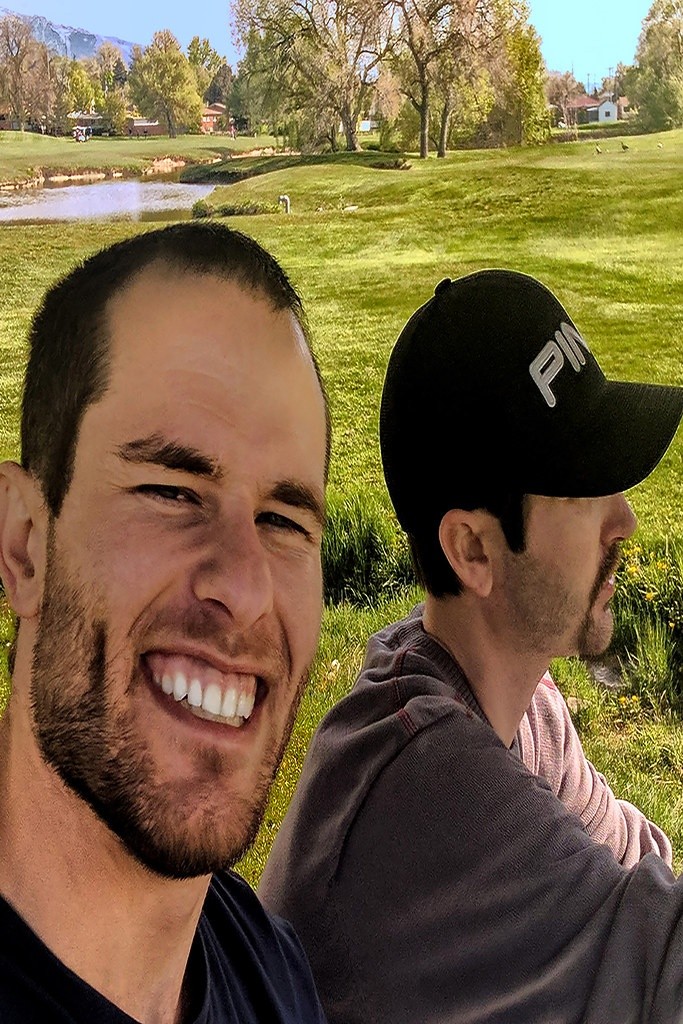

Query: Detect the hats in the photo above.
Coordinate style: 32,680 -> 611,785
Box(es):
380,267 -> 683,498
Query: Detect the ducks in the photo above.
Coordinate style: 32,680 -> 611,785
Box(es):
620,141 -> 629,151
595,139 -> 602,154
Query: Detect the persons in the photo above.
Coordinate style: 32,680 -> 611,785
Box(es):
252,268 -> 683,1024
0,222 -> 324,1024
